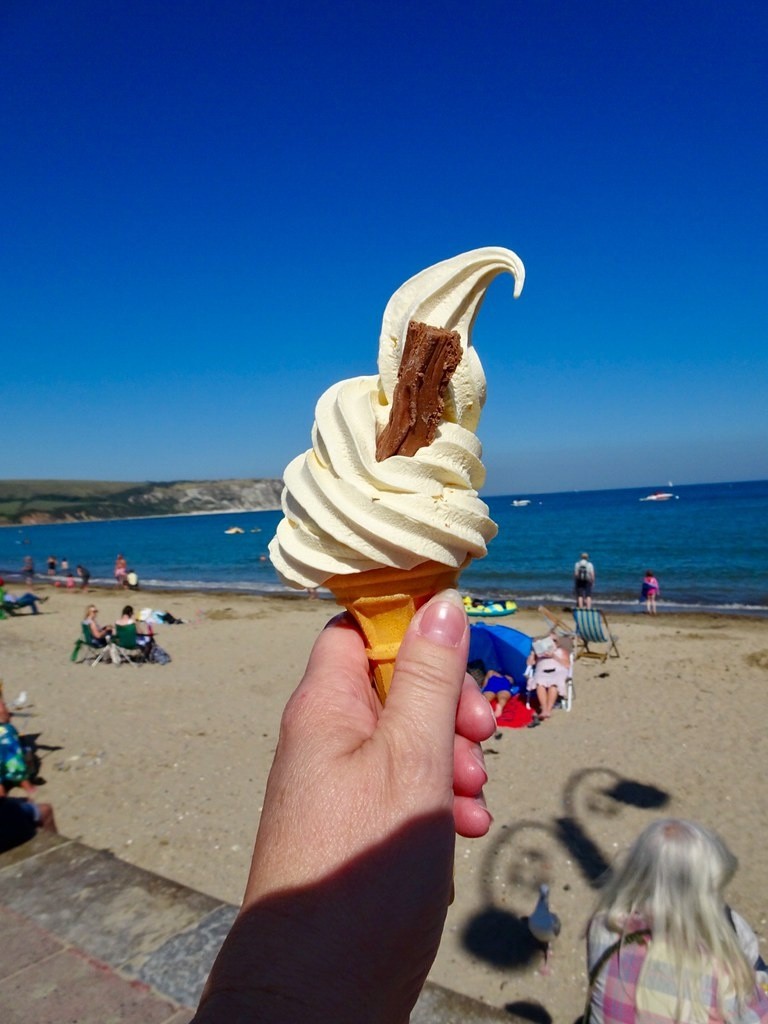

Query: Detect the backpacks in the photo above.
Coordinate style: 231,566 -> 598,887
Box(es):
577,563 -> 588,585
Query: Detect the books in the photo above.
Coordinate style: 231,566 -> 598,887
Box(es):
532,635 -> 558,658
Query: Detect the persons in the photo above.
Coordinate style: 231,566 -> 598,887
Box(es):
581,816 -> 768,1024
189,582 -> 492,1024
574,553 -> 595,610
525,631 -> 574,721
80,603 -> 116,649
0,795 -> 59,859
114,605 -> 156,664
480,668 -> 514,720
0,551 -> 138,616
0,682 -> 38,797
643,569 -> 661,616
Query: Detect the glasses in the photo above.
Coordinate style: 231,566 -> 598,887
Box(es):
92,610 -> 98,613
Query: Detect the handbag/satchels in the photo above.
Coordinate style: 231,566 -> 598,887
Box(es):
149,646 -> 171,665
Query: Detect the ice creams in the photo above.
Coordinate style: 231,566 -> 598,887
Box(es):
267,244 -> 524,710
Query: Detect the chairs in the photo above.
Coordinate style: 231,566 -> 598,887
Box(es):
0,589 -> 29,617
526,603 -> 620,711
78,623 -> 150,668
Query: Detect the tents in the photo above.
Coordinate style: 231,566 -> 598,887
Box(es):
464,620 -> 538,728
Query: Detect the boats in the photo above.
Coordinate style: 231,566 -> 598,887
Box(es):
639,493 -> 674,501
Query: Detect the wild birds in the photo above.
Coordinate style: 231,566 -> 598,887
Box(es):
7,691 -> 26,710
527,883 -> 561,978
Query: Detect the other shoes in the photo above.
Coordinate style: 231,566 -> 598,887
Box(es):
39,597 -> 48,604
